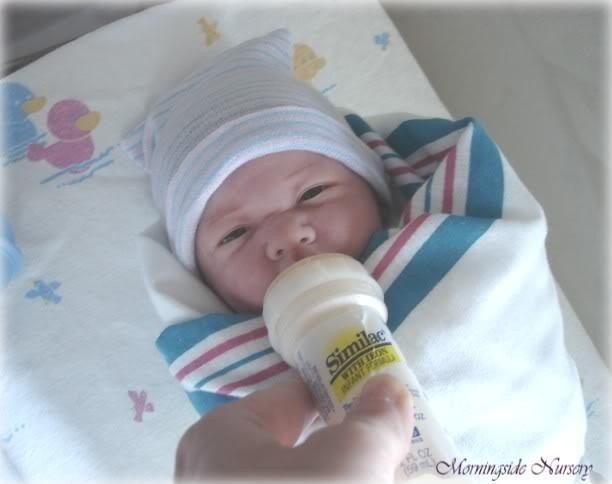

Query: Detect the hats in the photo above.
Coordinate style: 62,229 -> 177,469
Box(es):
119,28 -> 393,278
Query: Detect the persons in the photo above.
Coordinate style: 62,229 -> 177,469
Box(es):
173,373 -> 414,484
142,27 -> 384,317
